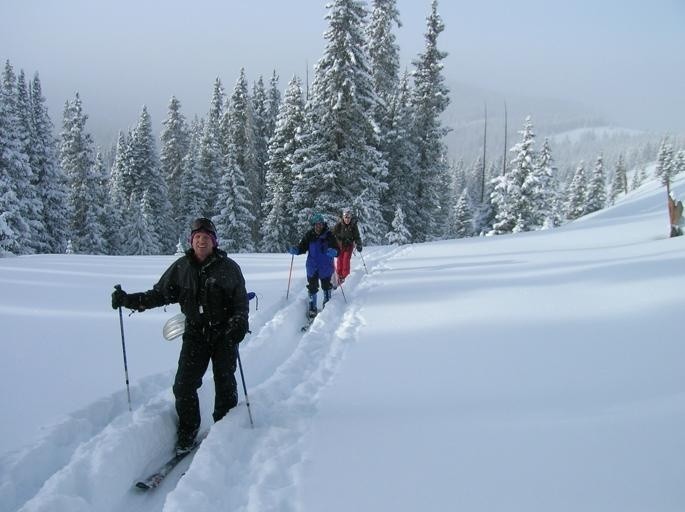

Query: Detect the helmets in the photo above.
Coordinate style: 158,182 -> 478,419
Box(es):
311,215 -> 325,226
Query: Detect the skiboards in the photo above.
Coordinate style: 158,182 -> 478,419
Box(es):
135,423 -> 214,490
301,315 -> 315,332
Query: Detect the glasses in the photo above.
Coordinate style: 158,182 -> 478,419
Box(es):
192,219 -> 216,238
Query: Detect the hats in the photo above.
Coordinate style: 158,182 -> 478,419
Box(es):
343,207 -> 353,217
191,218 -> 217,248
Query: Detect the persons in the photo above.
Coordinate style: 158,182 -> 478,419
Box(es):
334,211 -> 363,286
289,214 -> 340,324
111,218 -> 250,456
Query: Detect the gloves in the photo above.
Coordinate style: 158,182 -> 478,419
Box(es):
225,310 -> 249,347
112,285 -> 139,310
290,245 -> 300,255
356,243 -> 363,252
327,247 -> 338,258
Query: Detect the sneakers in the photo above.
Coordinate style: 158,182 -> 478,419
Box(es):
309,317 -> 314,324
175,428 -> 198,452
